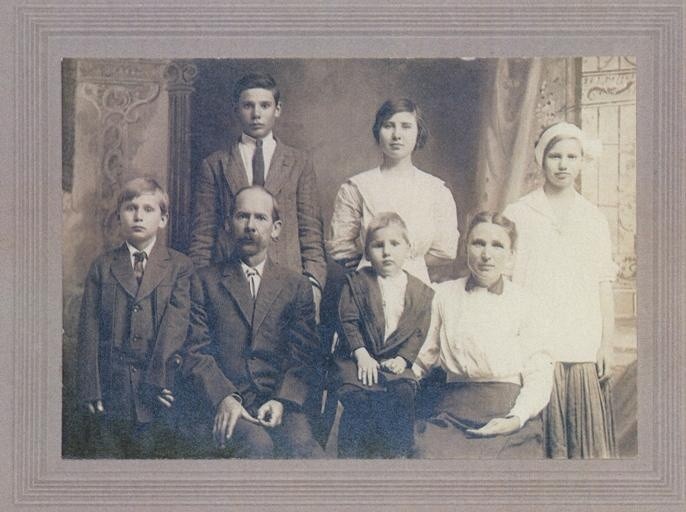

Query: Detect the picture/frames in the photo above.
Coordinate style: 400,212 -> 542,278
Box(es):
0,0 -> 686,512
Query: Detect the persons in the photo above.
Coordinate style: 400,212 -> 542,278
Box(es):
188,186 -> 325,460
74,174 -> 193,459
327,98 -> 460,285
502,119 -> 615,459
409,210 -> 553,459
187,71 -> 324,328
325,212 -> 434,460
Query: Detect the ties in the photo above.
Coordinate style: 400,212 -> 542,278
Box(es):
251,139 -> 265,186
245,269 -> 257,301
132,252 -> 147,287
